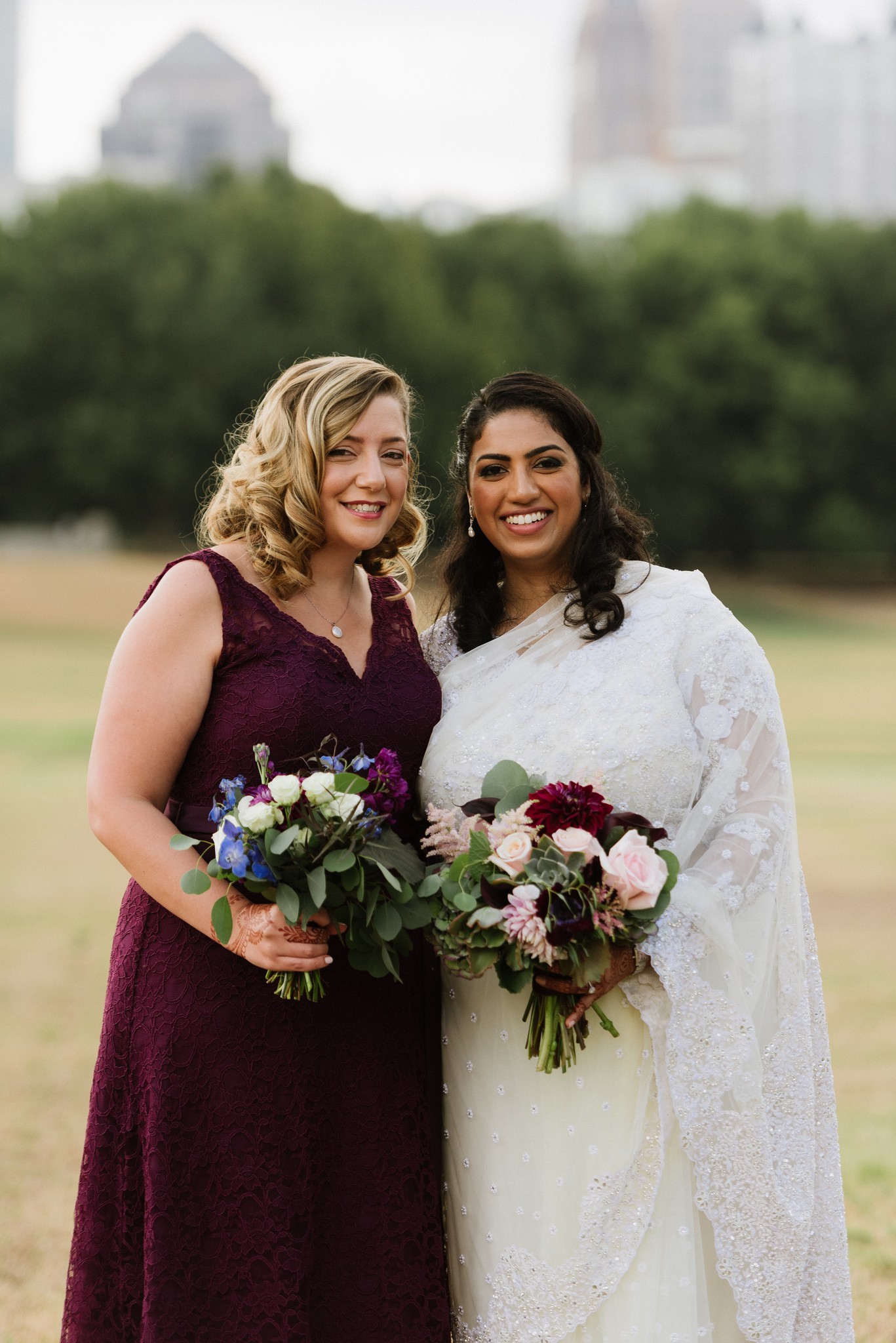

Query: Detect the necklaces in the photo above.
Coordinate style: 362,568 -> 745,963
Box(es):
499,580 -> 570,648
298,564 -> 355,637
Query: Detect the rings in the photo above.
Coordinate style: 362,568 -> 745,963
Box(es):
588,982 -> 596,995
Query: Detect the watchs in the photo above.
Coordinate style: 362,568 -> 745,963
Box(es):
629,942 -> 650,978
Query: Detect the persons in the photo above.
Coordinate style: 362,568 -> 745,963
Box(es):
86,355 -> 442,1342
418,373 -> 782,1343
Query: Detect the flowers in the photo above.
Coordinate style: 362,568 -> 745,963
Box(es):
170,741 -> 426,1007
417,757 -> 680,1076
